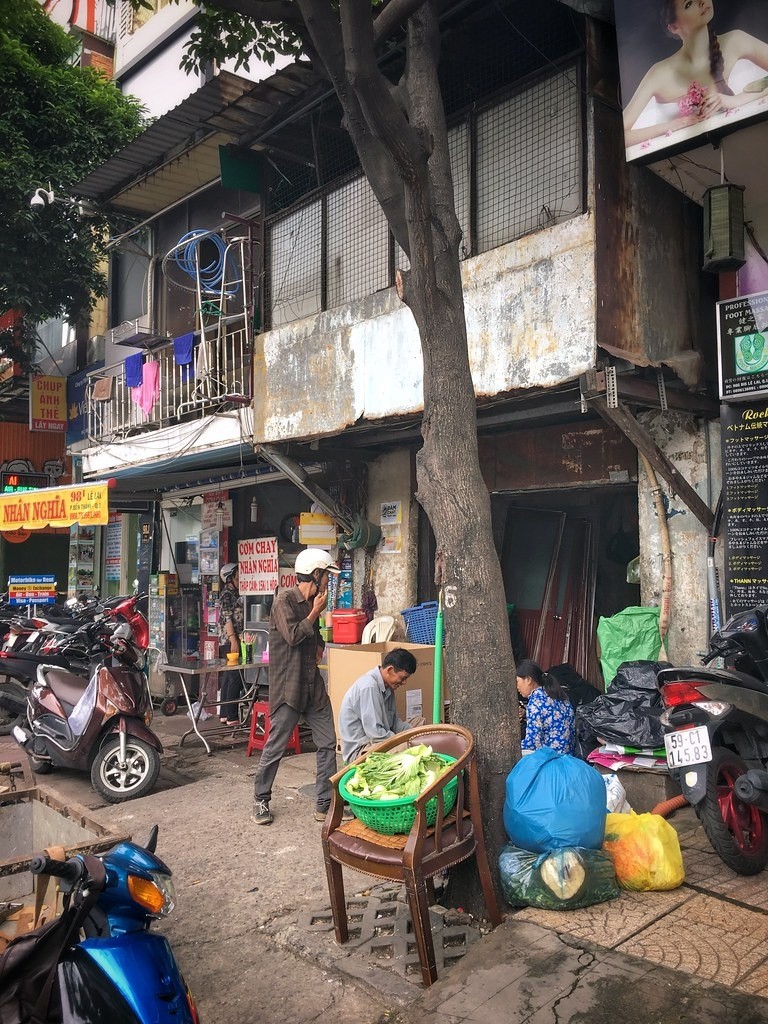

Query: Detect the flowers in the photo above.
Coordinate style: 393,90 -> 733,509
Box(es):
679,81 -> 728,117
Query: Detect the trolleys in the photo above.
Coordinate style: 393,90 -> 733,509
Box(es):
144,584 -> 200,717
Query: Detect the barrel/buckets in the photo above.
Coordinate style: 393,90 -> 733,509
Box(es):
251,604 -> 267,623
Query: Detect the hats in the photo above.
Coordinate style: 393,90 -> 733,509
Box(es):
326,566 -> 341,574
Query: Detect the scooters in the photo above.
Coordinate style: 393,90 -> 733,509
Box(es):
0,823 -> 203,1024
10,634 -> 164,803
656,603 -> 768,877
0,578 -> 150,736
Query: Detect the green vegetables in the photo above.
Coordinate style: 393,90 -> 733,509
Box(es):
344,742 -> 455,801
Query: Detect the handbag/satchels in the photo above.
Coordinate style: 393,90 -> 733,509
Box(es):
497,747 -> 684,910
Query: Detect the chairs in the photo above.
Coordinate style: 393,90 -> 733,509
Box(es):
321,724 -> 502,986
361,616 -> 396,644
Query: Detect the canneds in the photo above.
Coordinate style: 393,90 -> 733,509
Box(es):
325,611 -> 332,627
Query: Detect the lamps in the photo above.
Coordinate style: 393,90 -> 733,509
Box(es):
702,141 -> 747,274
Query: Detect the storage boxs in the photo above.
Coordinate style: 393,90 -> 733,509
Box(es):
327,641 -> 445,755
332,608 -> 367,643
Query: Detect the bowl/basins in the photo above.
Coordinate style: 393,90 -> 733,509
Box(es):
227,653 -> 239,661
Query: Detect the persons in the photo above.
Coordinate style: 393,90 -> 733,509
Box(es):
338,649 -> 417,765
517,659 -> 576,757
253,548 -> 353,825
622,0 -> 768,148
217,563 -> 244,724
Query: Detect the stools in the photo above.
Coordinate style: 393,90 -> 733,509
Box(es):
247,703 -> 301,758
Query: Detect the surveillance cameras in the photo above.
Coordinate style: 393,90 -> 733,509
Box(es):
31,195 -> 45,213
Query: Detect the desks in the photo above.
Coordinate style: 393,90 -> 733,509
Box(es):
158,661 -> 269,757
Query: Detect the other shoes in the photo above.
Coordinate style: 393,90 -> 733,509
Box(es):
222,716 -> 239,725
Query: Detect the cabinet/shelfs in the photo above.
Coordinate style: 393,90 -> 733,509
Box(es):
147,583 -> 201,716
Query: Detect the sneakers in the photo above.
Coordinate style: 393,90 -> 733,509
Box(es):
314,808 -> 356,822
251,799 -> 273,825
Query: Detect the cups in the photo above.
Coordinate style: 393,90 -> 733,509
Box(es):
241,644 -> 255,661
325,612 -> 332,628
319,618 -> 324,628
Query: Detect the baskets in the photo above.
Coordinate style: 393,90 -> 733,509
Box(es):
339,749 -> 464,833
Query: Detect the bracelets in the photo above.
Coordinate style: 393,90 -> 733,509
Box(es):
228,633 -> 235,639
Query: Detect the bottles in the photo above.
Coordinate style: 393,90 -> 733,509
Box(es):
167,597 -> 181,666
209,625 -> 218,633
201,552 -> 218,573
151,599 -> 164,652
204,575 -> 218,600
187,598 -> 200,656
183,584 -> 200,596
187,550 -> 198,567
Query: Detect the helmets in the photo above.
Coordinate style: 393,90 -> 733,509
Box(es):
294,549 -> 340,575
220,563 -> 238,583
